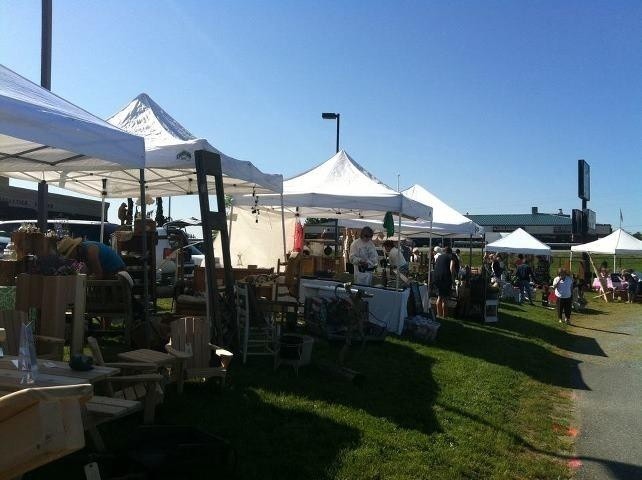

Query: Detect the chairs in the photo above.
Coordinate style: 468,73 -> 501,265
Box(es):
1,259 -> 298,480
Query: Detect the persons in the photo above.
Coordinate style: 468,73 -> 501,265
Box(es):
56,234 -> 127,328
596,260 -> 613,300
448,249 -> 460,276
432,245 -> 460,318
409,247 -> 422,280
454,247 -> 463,268
381,239 -> 409,289
574,251 -> 590,304
620,268 -> 639,305
551,266 -> 575,325
481,249 -> 552,307
432,245 -> 445,263
348,224 -> 380,287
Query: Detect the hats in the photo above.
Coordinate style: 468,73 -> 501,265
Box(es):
57,237 -> 82,256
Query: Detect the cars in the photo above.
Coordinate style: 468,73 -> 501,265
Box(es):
155,216 -> 205,298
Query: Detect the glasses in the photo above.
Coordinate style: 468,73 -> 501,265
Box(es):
365,235 -> 373,238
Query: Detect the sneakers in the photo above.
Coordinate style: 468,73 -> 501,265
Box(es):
559,319 -> 570,324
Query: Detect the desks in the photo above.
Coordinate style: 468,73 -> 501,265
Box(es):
299,278 -> 412,336
593,277 -> 629,300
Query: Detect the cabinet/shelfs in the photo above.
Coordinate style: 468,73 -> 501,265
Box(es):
110,230 -> 157,304
